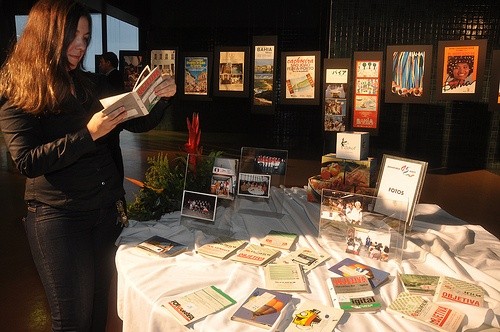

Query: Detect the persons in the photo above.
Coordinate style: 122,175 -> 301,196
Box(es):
448,56 -> 474,88
187,199 -> 210,213
257,155 -> 285,169
321,195 -> 389,261
0,0 -> 176,331
212,180 -> 230,195
241,181 -> 267,194
99,52 -> 124,146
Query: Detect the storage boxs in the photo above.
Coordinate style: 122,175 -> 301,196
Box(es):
306,131 -> 378,202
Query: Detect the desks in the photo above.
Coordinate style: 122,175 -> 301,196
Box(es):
115,185 -> 500,332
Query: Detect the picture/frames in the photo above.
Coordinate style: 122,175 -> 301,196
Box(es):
321,57 -> 353,133
384,45 -> 433,105
148,47 -> 178,83
179,168 -> 270,223
280,51 -> 320,107
349,51 -> 383,131
119,50 -> 146,76
251,34 -> 280,111
212,45 -> 250,97
487,49 -> 500,112
176,50 -> 214,101
434,38 -> 487,101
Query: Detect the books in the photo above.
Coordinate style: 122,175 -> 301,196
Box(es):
137,235 -> 188,258
99,65 -> 165,124
195,236 -> 246,259
230,229 -> 486,332
162,284 -> 236,327
